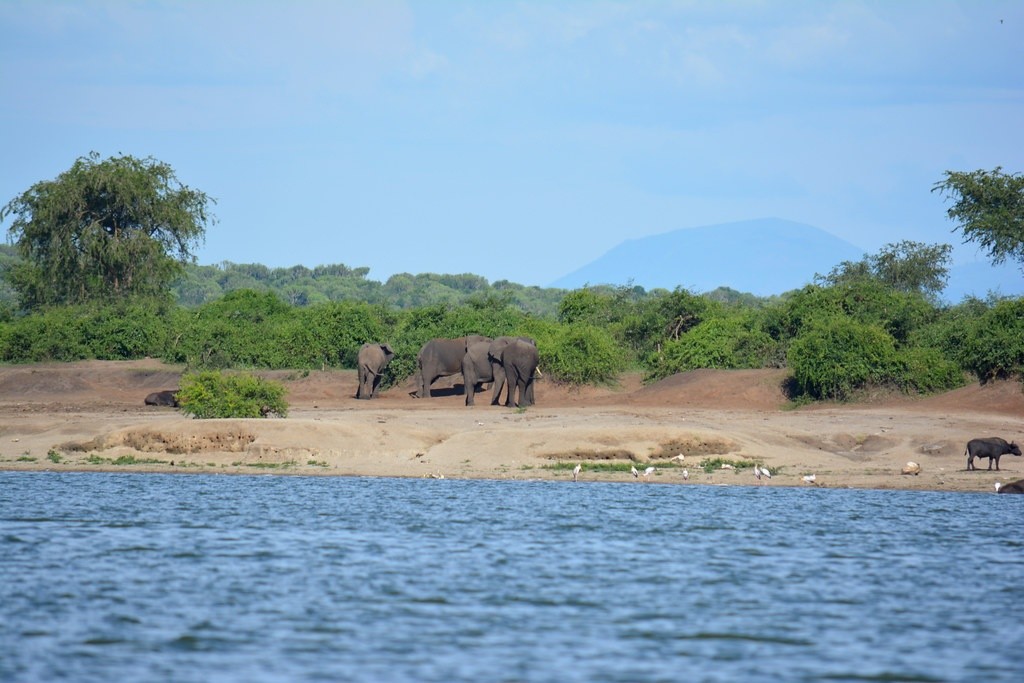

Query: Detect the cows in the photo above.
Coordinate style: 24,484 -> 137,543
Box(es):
964,437 -> 1022,471
998,479 -> 1024,495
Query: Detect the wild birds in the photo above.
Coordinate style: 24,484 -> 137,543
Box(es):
752,463 -> 772,481
572,463 -> 583,482
994,482 -> 1001,491
641,466 -> 658,481
669,452 -> 685,467
799,473 -> 818,486
629,465 -> 639,480
681,466 -> 689,481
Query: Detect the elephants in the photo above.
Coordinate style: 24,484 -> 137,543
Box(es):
355,335 -> 543,408
